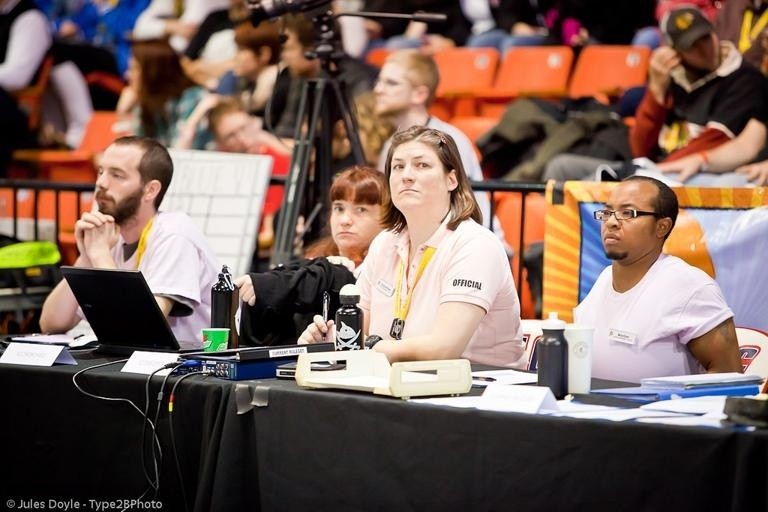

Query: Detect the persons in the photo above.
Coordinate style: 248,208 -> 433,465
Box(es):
0,1 -> 768,385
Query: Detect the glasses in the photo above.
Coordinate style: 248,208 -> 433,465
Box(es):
593,209 -> 661,221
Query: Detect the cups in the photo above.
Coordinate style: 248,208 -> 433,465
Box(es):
200,328 -> 230,352
562,322 -> 593,396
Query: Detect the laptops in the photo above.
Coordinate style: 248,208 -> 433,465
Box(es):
60,266 -> 205,359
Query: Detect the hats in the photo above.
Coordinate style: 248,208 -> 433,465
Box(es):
667,8 -> 713,50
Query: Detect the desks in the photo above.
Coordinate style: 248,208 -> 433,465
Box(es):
0,335 -> 763,508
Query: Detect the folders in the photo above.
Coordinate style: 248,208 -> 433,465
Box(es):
180,343 -> 336,362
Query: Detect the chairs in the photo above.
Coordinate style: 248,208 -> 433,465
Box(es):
0,45 -> 669,318
705,326 -> 767,393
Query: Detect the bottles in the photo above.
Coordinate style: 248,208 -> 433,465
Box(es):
536,310 -> 569,397
212,265 -> 241,351
335,284 -> 365,354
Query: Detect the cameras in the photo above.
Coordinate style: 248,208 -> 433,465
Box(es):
247,0 -> 335,29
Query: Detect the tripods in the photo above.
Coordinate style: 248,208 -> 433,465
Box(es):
268,76 -> 367,273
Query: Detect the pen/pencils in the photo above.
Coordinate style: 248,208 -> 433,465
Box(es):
322,291 -> 330,342
473,376 -> 497,382
